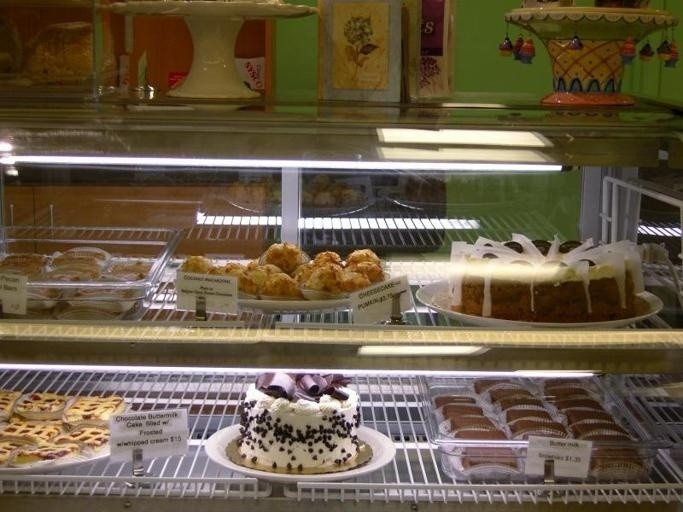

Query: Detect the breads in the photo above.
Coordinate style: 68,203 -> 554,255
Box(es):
0,389 -> 125,468
0,13 -> 19,72
22,21 -> 114,84
1,250 -> 149,313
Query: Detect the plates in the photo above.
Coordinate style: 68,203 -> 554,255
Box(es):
206,423 -> 398,481
237,271 -> 390,312
2,393 -> 115,475
426,280 -> 665,329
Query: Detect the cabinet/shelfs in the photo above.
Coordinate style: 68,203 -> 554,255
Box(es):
2,160 -> 681,509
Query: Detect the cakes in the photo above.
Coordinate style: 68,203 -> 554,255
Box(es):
236,372 -> 363,469
449,232 -> 646,322
435,377 -> 644,472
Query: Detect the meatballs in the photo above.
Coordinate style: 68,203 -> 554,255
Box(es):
185,242 -> 385,300
228,174 -> 366,210
